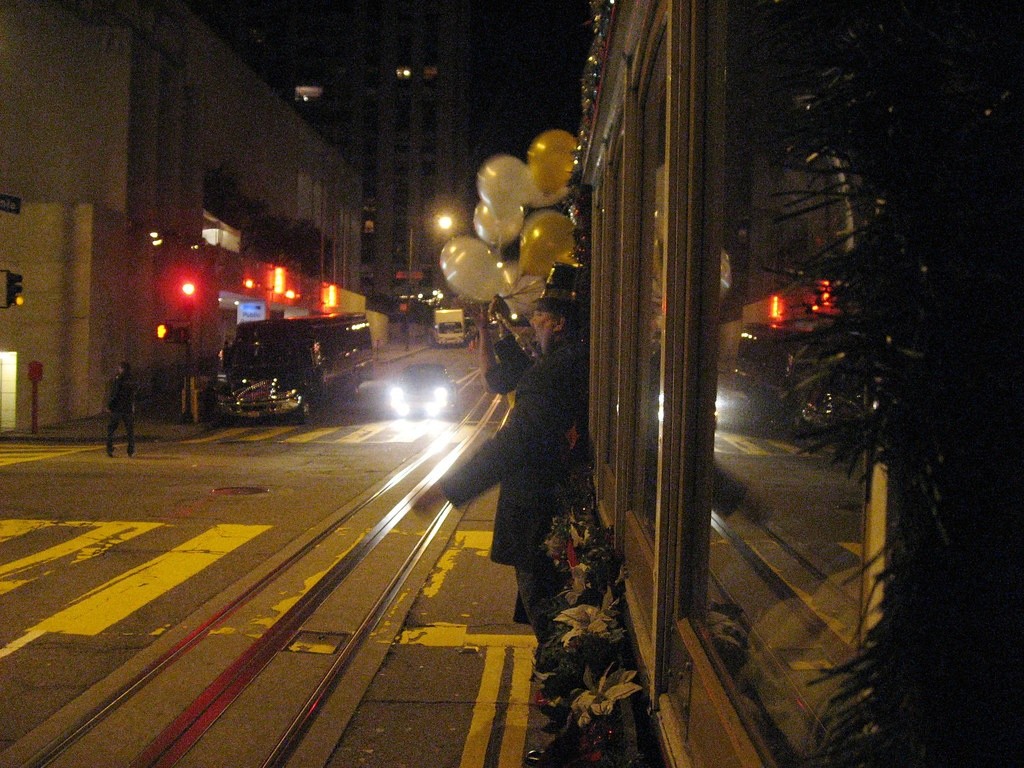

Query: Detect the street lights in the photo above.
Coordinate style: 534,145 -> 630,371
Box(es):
406,216 -> 454,317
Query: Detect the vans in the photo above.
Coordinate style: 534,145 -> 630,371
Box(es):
213,311 -> 376,425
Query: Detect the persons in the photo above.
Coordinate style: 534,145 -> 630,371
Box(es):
105,362 -> 137,458
414,265 -> 591,708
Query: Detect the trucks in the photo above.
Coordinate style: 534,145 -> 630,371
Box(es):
435,309 -> 466,348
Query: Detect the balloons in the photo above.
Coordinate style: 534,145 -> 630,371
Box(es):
438,129 -> 576,312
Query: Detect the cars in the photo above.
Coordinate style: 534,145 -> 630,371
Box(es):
715,323 -> 861,443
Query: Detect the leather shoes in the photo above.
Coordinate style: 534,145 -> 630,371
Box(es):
525,748 -> 554,765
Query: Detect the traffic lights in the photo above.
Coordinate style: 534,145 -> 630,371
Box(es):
5,269 -> 23,309
156,323 -> 170,340
